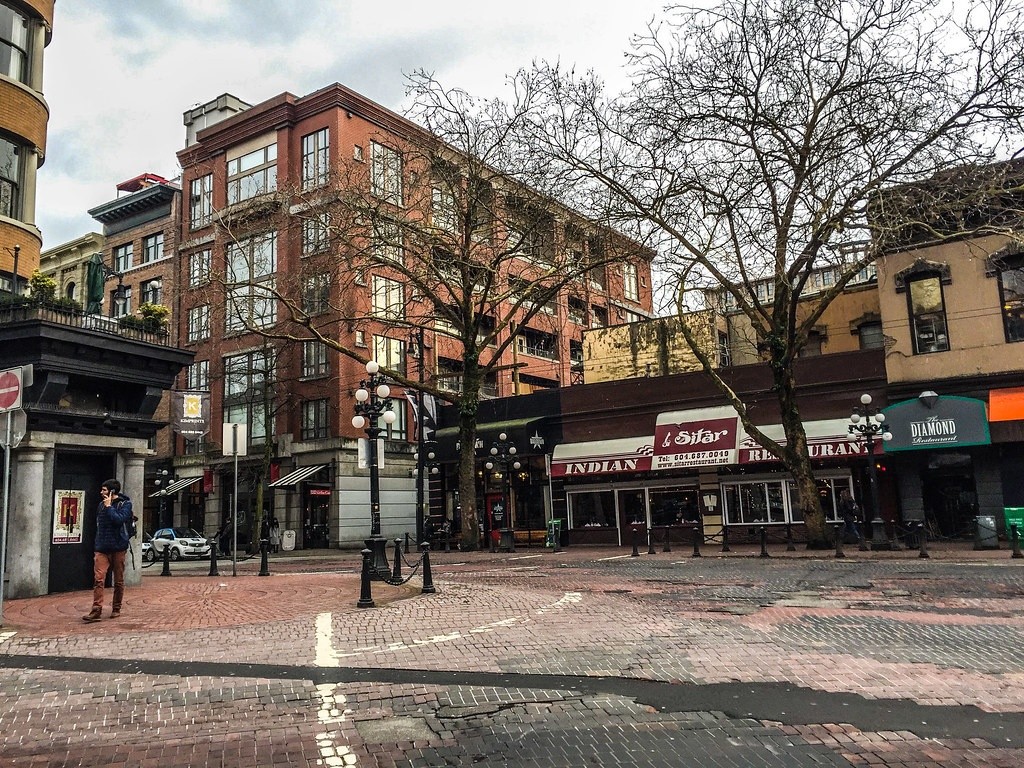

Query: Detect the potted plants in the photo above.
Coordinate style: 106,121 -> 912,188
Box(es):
0,269 -> 83,328
118,302 -> 172,344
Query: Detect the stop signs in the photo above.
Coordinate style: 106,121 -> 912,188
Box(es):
0,365 -> 23,413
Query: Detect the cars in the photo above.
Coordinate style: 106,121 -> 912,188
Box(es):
142,531 -> 153,558
146,526 -> 212,561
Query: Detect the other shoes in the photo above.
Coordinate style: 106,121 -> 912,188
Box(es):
82,606 -> 102,621
110,607 -> 120,618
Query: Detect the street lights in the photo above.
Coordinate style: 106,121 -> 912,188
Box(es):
487,433 -> 522,554
847,394 -> 895,551
353,360 -> 397,582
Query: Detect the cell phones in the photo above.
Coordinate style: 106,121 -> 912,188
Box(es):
108,490 -> 115,497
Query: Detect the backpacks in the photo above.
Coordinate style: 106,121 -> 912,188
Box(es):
117,500 -> 139,539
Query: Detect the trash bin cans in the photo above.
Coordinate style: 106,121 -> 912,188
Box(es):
976,515 -> 998,547
1002,506 -> 1024,548
903,519 -> 925,549
500,528 -> 514,549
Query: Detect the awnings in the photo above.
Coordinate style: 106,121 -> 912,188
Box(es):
550,401 -> 885,478
882,386 -> 1024,452
434,415 -> 555,439
148,477 -> 204,497
266,463 -> 328,489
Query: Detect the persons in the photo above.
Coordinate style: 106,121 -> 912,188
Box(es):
839,487 -> 861,541
752,514 -> 764,522
212,525 -> 225,554
260,517 -> 280,553
219,517 -> 233,556
82,478 -> 134,623
